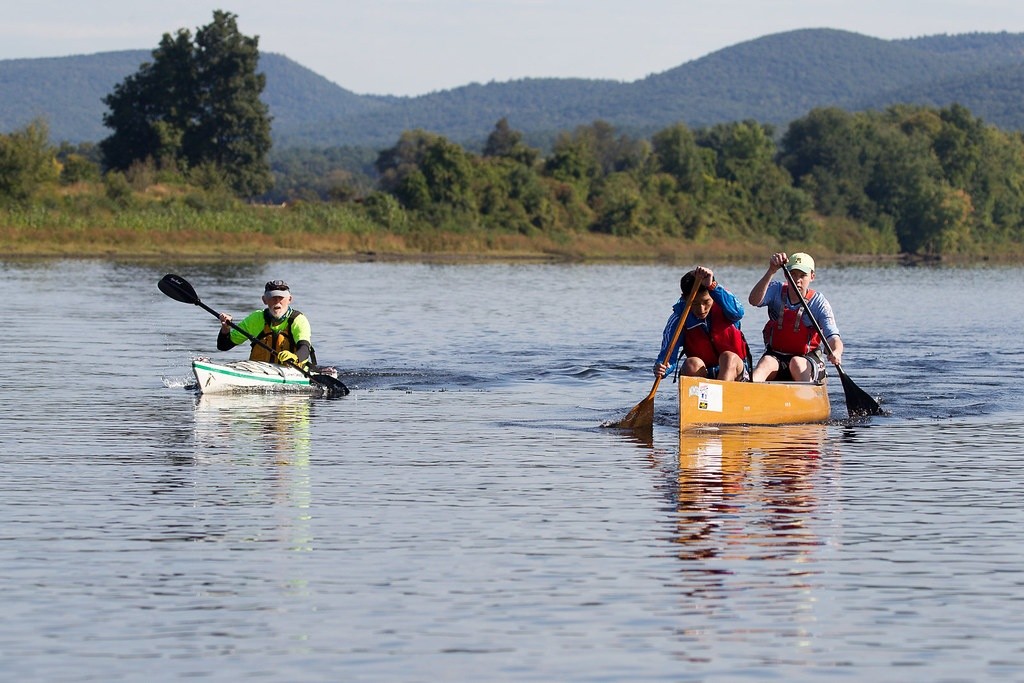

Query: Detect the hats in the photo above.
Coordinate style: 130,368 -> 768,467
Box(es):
264,280 -> 291,298
785,252 -> 815,274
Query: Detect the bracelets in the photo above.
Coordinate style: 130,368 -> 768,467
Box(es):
708,281 -> 717,290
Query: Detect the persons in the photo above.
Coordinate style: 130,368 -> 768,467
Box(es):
218,279 -> 311,370
653,267 -> 752,383
748,253 -> 844,383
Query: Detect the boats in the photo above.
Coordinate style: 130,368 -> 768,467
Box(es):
191,360 -> 339,396
676,376 -> 833,432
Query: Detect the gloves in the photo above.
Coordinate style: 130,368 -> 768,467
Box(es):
278,350 -> 298,365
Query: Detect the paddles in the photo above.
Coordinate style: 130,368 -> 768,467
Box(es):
781,263 -> 884,415
157,273 -> 350,392
620,274 -> 702,429
